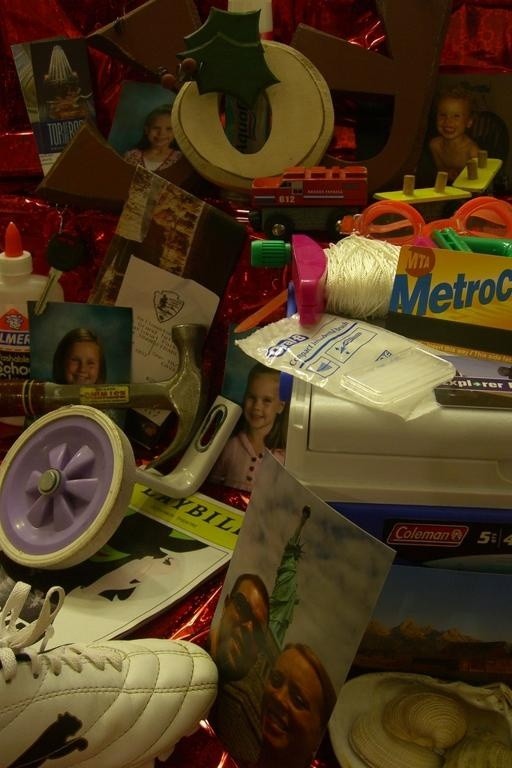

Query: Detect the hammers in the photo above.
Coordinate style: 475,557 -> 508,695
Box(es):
0,323 -> 206,470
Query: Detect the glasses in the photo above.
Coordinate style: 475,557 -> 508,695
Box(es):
232,591 -> 265,647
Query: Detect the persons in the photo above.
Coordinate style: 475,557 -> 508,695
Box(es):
428,83 -> 485,181
52,326 -> 107,387
122,103 -> 185,173
261,504 -> 313,648
207,361 -> 291,492
252,642 -> 337,768
0,510 -> 209,650
205,570 -> 277,768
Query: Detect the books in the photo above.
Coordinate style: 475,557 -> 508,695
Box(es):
0,468 -> 246,652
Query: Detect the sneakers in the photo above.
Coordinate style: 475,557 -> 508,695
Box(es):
0,581 -> 217,768
